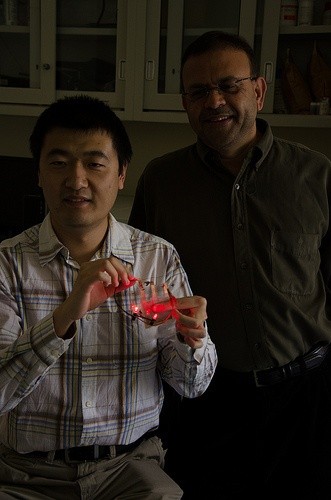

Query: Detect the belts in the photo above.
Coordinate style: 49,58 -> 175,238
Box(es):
30,427 -> 159,458
234,342 -> 329,388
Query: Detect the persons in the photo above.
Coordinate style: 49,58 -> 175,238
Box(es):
128,32 -> 331,500
0,95 -> 217,500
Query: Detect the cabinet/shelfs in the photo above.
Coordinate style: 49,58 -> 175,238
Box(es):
0,0 -> 331,127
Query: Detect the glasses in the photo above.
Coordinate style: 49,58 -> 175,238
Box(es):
179,76 -> 250,99
